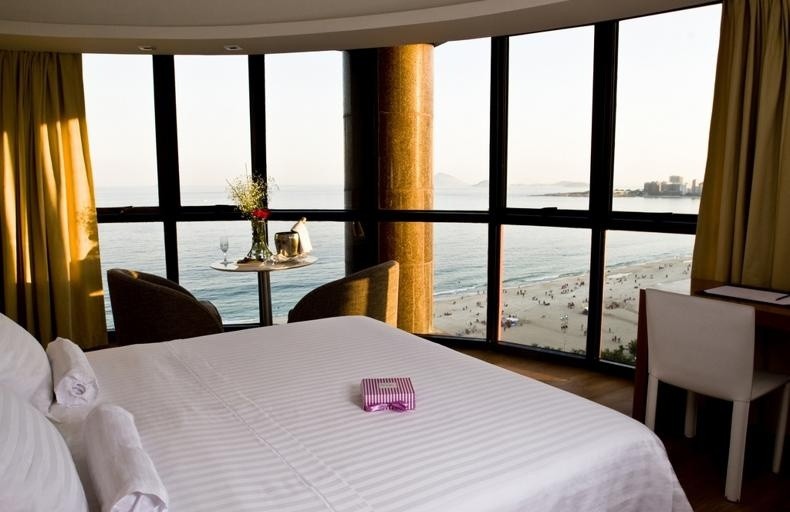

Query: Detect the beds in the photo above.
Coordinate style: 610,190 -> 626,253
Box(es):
42,313 -> 694,511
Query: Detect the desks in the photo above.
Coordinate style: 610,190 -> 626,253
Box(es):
210,254 -> 318,326
630,276 -> 790,436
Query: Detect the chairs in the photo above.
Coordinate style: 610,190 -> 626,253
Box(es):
288,258 -> 399,330
107,268 -> 226,347
645,288 -> 790,502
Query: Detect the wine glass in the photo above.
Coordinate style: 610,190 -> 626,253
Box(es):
220,236 -> 232,264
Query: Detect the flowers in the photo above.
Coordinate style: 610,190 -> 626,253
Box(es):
228,170 -> 281,259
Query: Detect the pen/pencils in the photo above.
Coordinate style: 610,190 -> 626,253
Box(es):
776,294 -> 790,301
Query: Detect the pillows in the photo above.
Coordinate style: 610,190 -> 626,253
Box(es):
0,311 -> 170,511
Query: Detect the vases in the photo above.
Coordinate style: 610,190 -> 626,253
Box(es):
235,220 -> 273,264
